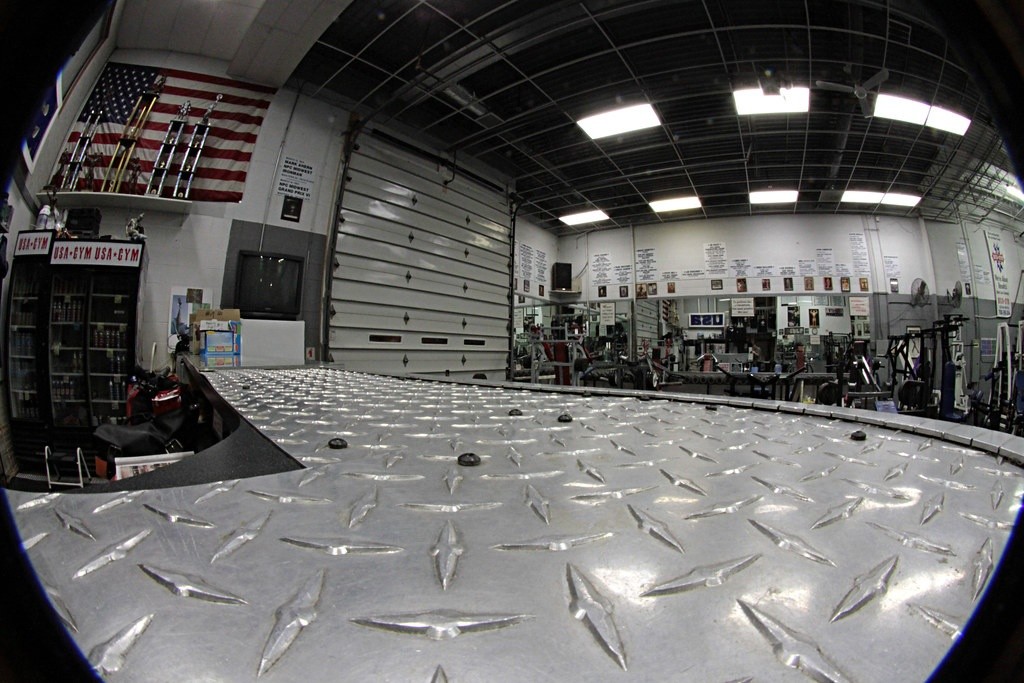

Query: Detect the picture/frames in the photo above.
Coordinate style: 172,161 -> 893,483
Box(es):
952,341 -> 965,367
906,326 -> 922,359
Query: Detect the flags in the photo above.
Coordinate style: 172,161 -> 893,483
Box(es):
49,58 -> 277,203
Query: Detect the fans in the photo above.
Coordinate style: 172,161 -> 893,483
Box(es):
910,278 -> 929,308
946,280 -> 962,309
815,67 -> 889,119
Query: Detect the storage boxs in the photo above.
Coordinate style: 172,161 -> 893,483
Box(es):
199,354 -> 241,368
198,320 -> 241,355
188,309 -> 240,355
240,318 -> 305,367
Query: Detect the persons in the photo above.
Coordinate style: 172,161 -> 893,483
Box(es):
750,343 -> 764,372
125,212 -> 148,240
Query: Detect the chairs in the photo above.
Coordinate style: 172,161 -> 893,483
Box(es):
898,381 -> 926,411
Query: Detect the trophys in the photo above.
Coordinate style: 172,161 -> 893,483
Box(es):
58,94 -> 223,196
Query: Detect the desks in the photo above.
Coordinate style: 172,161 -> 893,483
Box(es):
669,371 -> 850,400
715,353 -> 753,373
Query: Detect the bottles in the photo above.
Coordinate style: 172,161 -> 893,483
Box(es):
108,380 -> 127,400
53,380 -> 76,401
52,300 -> 84,321
12,277 -> 39,419
93,329 -> 126,373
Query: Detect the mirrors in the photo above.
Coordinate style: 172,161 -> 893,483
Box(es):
634,292 -> 877,373
560,298 -> 633,362
514,292 -> 559,370
